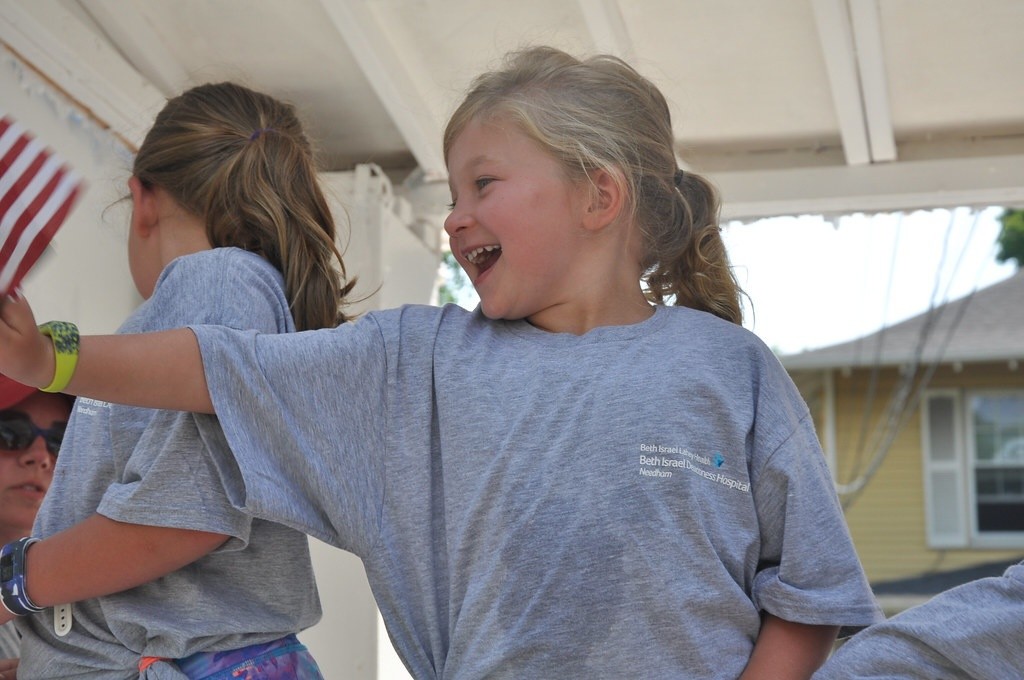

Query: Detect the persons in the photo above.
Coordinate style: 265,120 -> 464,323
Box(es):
1,374 -> 77,660
0,44 -> 852,680
0,83 -> 346,680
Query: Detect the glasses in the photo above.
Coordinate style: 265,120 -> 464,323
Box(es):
0,409 -> 69,457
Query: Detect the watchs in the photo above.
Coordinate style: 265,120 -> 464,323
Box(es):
37,320 -> 79,394
0,536 -> 73,637
